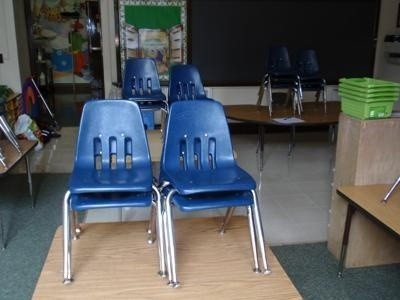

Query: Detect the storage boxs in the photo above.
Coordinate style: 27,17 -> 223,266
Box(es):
338,76 -> 400,120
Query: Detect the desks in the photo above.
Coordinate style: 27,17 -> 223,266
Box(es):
38,66 -> 55,120
0,138 -> 38,250
336,183 -> 400,279
31,217 -> 303,299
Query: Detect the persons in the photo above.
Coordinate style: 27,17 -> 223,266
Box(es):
88,11 -> 102,80
68,21 -> 89,77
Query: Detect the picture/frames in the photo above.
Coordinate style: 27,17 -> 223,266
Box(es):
23,0 -> 92,93
114,0 -> 192,88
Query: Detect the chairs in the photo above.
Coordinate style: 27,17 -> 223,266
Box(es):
0,109 -> 21,168
63,99 -> 169,286
381,176 -> 400,203
157,97 -> 270,286
116,56 -> 208,127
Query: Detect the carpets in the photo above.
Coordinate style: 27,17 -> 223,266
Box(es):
269,241 -> 400,300
0,171 -> 87,300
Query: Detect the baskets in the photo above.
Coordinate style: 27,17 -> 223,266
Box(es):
338,78 -> 400,119
